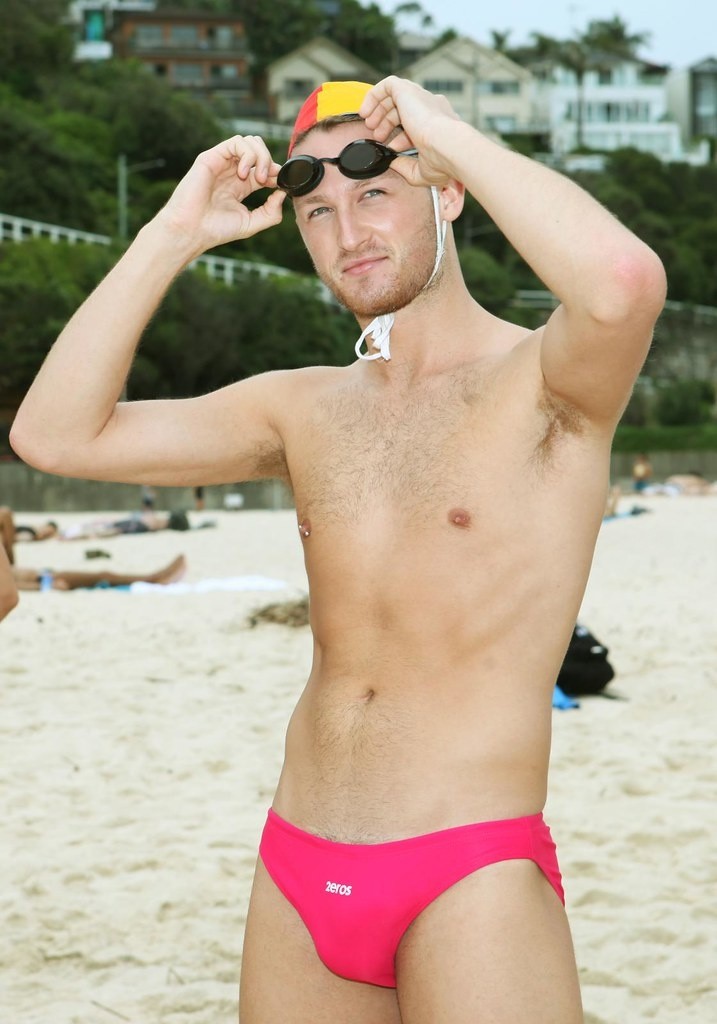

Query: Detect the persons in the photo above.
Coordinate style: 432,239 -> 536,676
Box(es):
0,484 -> 244,623
602,453 -> 717,521
10,74 -> 668,1024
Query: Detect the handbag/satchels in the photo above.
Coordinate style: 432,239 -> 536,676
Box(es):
169,509 -> 189,530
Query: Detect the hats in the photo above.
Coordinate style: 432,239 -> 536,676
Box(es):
286,81 -> 376,157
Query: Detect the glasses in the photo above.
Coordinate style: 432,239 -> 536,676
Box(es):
277,138 -> 418,197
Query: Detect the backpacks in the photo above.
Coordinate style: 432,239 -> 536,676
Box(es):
557,623 -> 613,697
